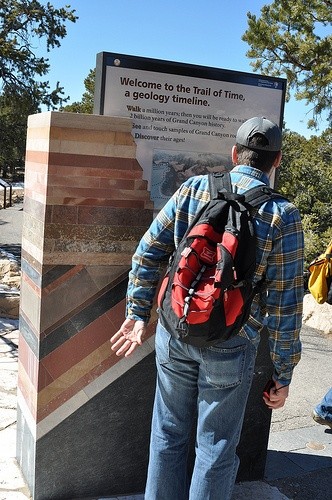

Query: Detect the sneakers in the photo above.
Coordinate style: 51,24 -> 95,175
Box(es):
311,409 -> 332,429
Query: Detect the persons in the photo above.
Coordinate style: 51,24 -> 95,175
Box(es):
109,116 -> 305,500
307,219 -> 332,429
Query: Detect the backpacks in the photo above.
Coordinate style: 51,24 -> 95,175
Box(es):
156,170 -> 289,348
308,239 -> 332,304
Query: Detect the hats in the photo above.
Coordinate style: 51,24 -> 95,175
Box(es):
236,116 -> 283,151
